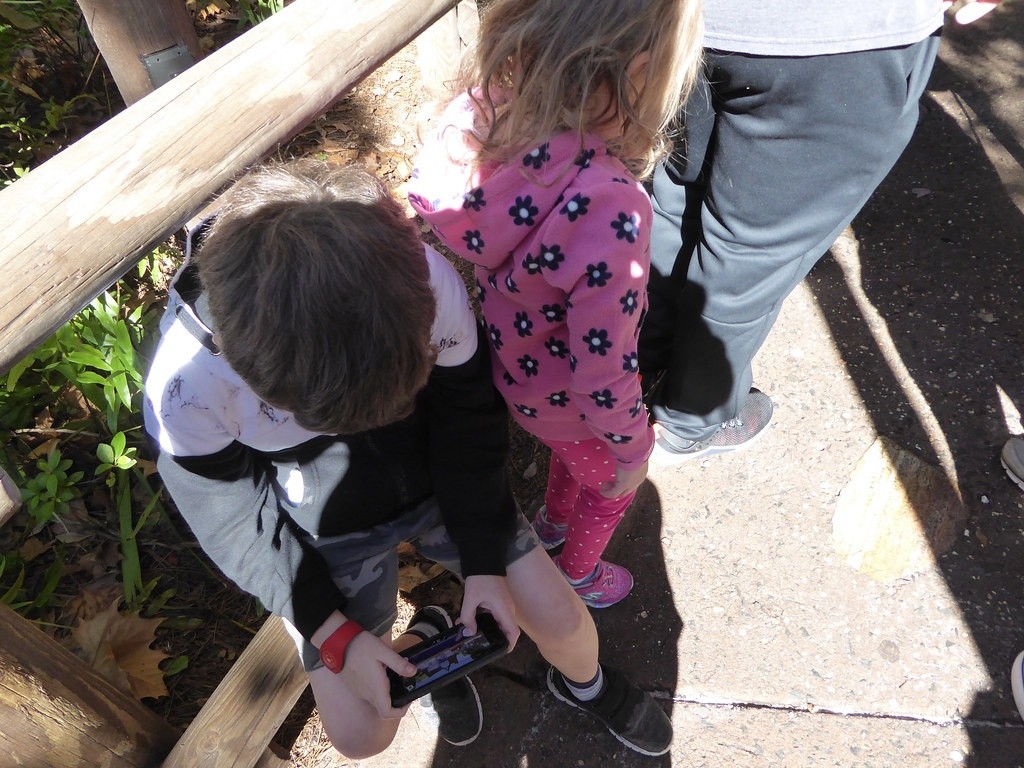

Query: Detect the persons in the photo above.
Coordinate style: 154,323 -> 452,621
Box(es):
643,0 -> 953,467
142,156 -> 676,758
404,0 -> 703,610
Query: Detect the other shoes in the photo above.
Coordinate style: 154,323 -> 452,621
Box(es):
1001,437 -> 1024,491
1011,651 -> 1024,721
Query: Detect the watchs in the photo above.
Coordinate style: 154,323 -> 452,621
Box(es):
319,620 -> 365,675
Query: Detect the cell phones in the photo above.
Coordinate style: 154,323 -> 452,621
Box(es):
388,612 -> 508,710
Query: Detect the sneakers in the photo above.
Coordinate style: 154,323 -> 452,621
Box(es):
553,554 -> 631,607
407,606 -> 484,747
548,659 -> 674,756
532,504 -> 569,552
650,389 -> 775,469
